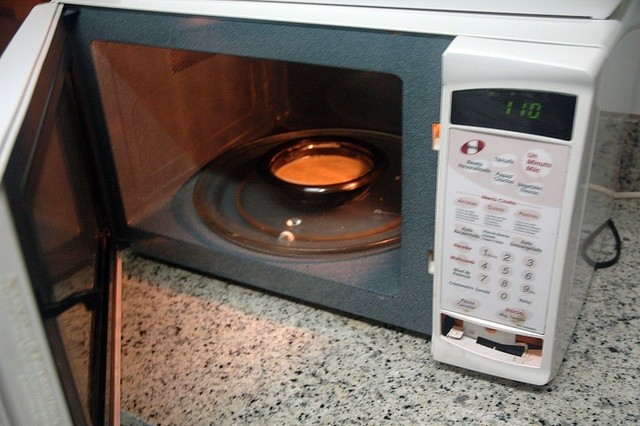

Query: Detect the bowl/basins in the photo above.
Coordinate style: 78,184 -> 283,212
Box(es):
257,133 -> 383,210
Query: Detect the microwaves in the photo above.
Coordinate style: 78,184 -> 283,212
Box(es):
1,0 -> 637,426
